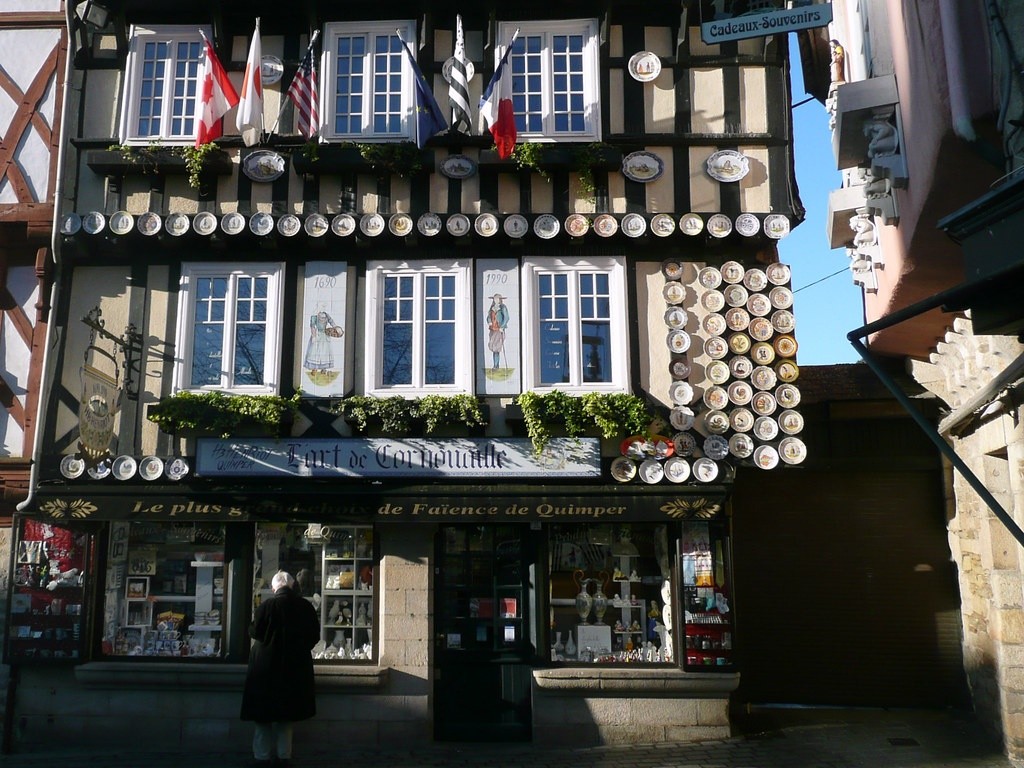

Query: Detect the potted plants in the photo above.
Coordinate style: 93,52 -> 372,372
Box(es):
330,394 -> 489,434
293,141 -> 422,186
506,388 -> 671,454
479,140 -> 621,205
86,142 -> 222,189
147,389 -> 301,443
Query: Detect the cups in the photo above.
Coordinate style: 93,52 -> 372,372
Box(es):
688,656 -> 726,665
144,630 -> 183,657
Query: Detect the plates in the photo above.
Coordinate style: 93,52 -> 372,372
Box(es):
439,154 -> 478,179
59,453 -> 191,482
260,54 -> 284,85
628,51 -> 662,82
58,210 -> 246,236
242,150 -> 285,184
621,150 -> 665,183
441,56 -> 475,85
248,212 -> 793,239
610,256 -> 809,485
706,149 -> 750,184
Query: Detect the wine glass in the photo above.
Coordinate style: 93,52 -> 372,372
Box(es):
184,634 -> 210,657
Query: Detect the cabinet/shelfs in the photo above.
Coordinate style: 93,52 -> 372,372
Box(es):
675,539 -> 734,672
119,577 -> 153,649
188,561 -> 223,630
3,512 -> 101,663
612,554 -> 641,650
321,525 -> 373,650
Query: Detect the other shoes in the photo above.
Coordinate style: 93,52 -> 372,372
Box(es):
245,758 -> 271,768
272,755 -> 288,768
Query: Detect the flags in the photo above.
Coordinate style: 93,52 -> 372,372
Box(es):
448,19 -> 472,136
408,53 -> 448,150
478,38 -> 518,160
194,39 -> 239,149
287,41 -> 320,142
234,27 -> 263,147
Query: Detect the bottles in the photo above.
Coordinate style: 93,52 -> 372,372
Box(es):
550,629 -> 670,663
686,633 -> 728,649
115,630 -> 127,654
311,629 -> 373,660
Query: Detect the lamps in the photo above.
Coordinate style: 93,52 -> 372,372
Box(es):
75,0 -> 111,29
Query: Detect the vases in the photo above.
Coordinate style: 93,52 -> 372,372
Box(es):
554,632 -> 564,652
576,578 -> 593,626
345,638 -> 351,651
591,578 -> 608,625
565,631 -> 576,654
333,630 -> 345,647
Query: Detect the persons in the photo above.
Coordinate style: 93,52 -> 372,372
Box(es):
858,169 -> 886,198
239,571 -> 321,762
846,247 -> 866,272
297,570 -> 321,621
861,119 -> 895,158
828,39 -> 845,81
850,218 -> 873,246
